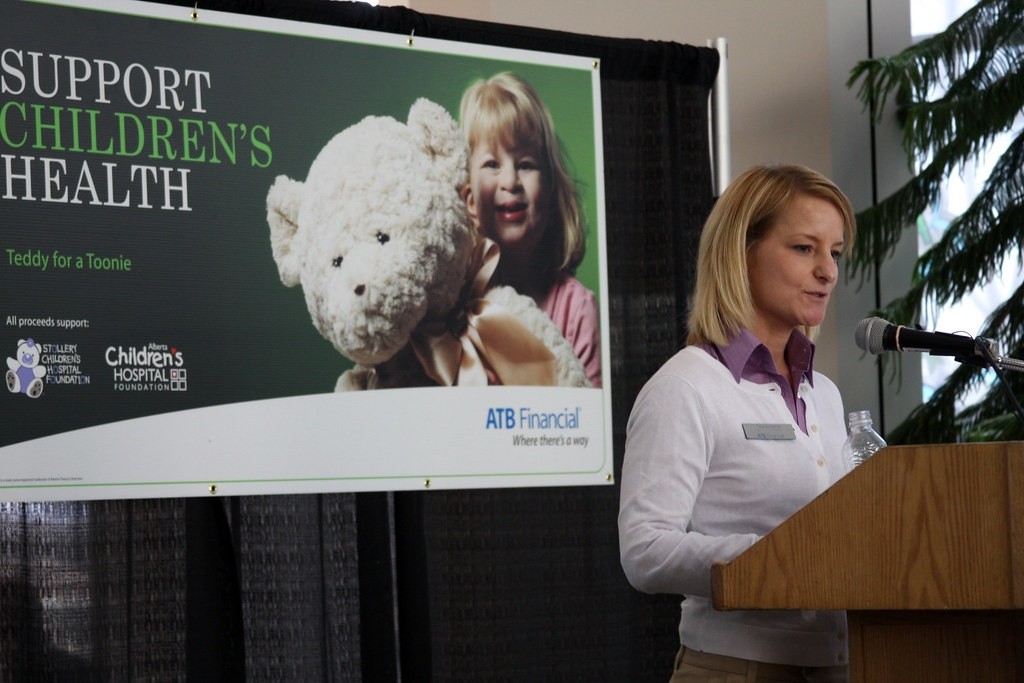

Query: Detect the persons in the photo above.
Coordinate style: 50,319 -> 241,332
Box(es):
617,164 -> 855,683
458,69 -> 603,388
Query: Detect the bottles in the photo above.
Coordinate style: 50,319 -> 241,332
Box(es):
841,410 -> 887,471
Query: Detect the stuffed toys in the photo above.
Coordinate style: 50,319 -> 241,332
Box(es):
266,96 -> 593,393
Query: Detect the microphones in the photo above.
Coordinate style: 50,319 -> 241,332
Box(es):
854,317 -> 999,359
